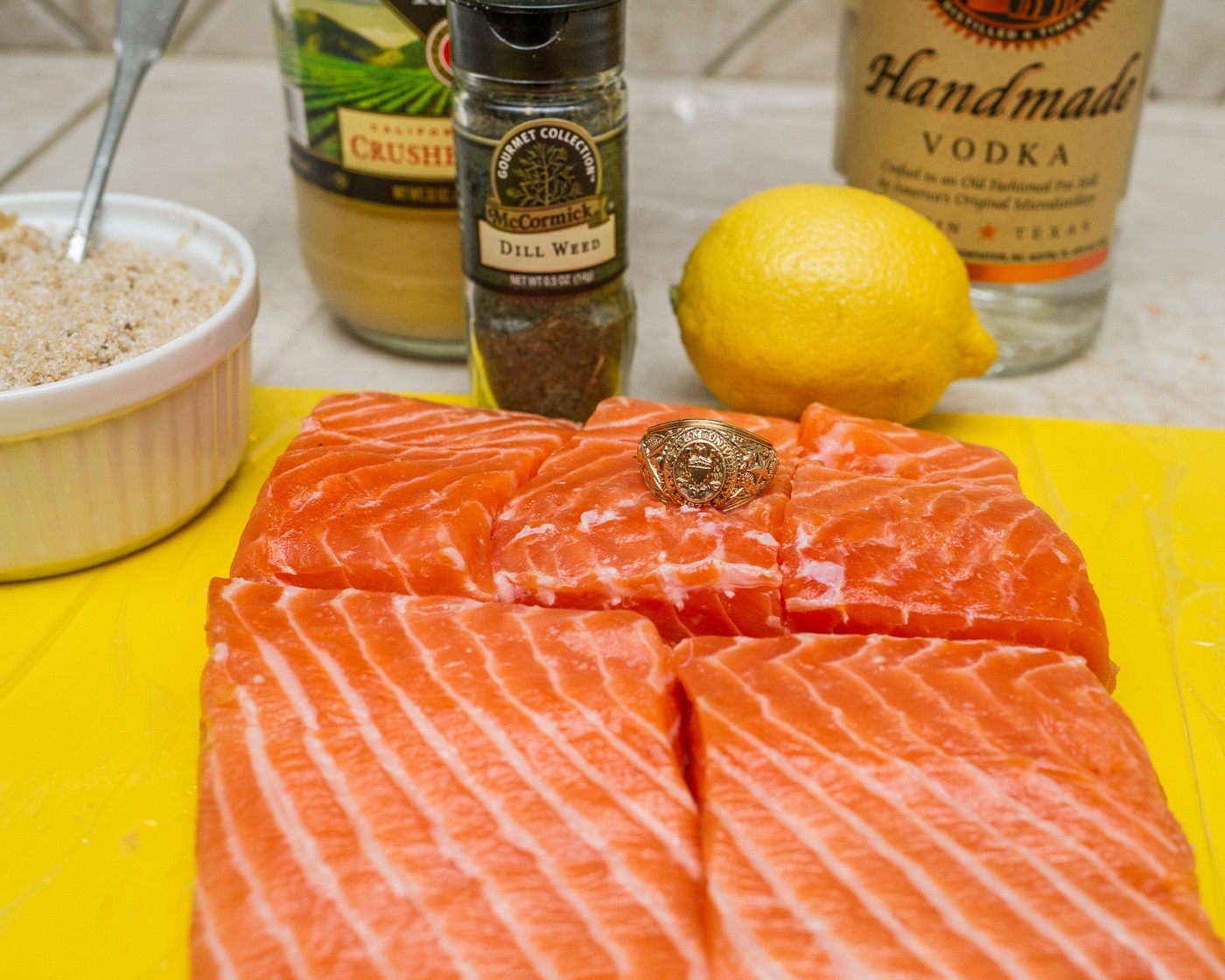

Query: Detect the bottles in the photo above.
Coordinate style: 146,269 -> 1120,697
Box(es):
833,0 -> 1161,377
450,0 -> 637,428
270,0 -> 471,358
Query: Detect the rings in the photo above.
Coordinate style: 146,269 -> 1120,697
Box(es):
637,418 -> 778,512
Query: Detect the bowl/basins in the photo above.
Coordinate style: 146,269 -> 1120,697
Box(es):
0,192 -> 257,583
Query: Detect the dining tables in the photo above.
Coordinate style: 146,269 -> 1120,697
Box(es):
0,51 -> 1224,979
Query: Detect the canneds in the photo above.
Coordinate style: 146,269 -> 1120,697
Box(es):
833,0 -> 1164,377
270,0 -> 469,361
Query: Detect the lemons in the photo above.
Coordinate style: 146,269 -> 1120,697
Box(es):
669,183 -> 999,423
